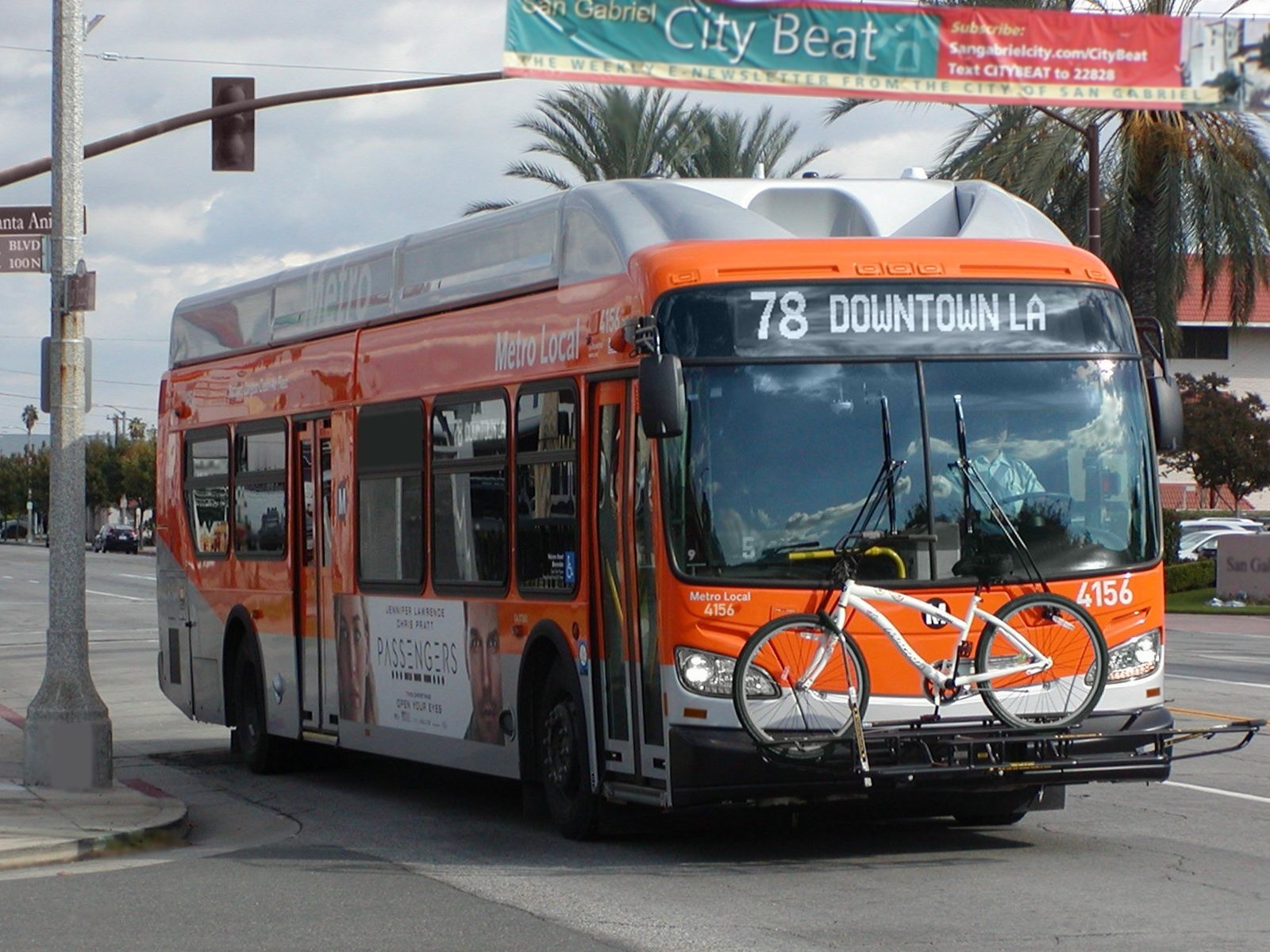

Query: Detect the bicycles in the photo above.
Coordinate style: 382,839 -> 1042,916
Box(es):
730,530 -> 1112,765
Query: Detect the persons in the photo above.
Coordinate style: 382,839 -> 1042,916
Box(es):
935,402 -> 1050,534
334,593 -> 376,726
462,601 -> 504,745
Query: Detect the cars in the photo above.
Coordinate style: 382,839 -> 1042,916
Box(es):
0,519 -> 29,539
92,523 -> 140,554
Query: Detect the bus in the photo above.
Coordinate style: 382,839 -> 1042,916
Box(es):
156,165 -> 1265,850
